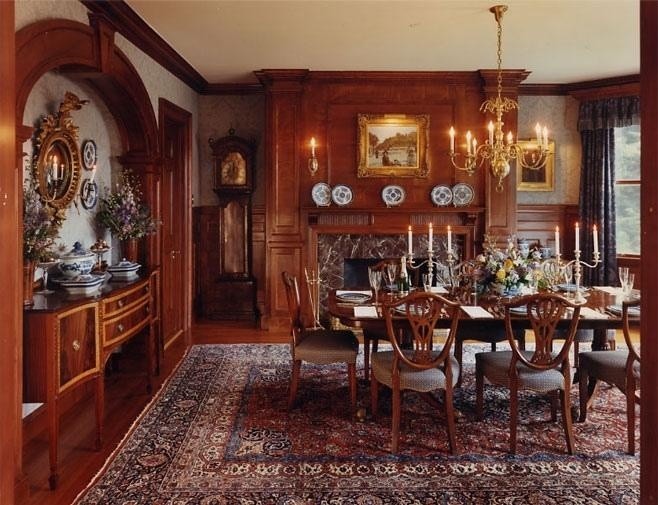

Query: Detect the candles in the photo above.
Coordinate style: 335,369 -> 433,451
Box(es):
52,156 -> 59,181
61,164 -> 64,180
574,222 -> 580,251
554,226 -> 561,255
407,225 -> 414,255
593,224 -> 599,252
447,225 -> 452,252
428,222 -> 433,253
90,164 -> 97,183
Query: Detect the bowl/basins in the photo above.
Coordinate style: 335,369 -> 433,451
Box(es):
519,245 -> 530,251
541,249 -> 551,253
542,253 -> 553,260
519,241 -> 529,244
519,251 -> 530,259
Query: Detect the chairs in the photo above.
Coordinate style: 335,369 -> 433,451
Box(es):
474,294 -> 581,457
281,270 -> 358,411
369,290 -> 460,456
368,259 -> 420,351
578,299 -> 640,456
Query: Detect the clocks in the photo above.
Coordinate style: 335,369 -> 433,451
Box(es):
208,127 -> 257,283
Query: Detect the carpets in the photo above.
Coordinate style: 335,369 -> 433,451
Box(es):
66,342 -> 640,504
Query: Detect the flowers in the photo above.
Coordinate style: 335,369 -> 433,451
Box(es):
21,156 -> 69,262
473,236 -> 558,295
97,169 -> 164,238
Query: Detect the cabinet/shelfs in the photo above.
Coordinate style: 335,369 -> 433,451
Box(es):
24,267 -> 160,491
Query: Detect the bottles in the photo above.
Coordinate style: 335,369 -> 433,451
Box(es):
397,255 -> 409,298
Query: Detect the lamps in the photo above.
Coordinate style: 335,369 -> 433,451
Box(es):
448,4 -> 553,189
309,137 -> 318,175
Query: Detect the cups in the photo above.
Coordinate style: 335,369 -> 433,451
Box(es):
382,264 -> 388,290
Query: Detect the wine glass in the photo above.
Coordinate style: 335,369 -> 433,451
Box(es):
387,264 -> 397,295
368,265 -> 382,305
529,274 -> 538,295
618,267 -> 629,288
422,274 -> 434,292
565,265 -> 575,299
625,274 -> 636,303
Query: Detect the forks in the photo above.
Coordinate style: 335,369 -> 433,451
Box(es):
488,307 -> 499,318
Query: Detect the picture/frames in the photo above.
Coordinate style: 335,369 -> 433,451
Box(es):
516,139 -> 556,191
357,113 -> 431,178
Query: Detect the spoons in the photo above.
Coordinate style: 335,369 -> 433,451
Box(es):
494,306 -> 505,317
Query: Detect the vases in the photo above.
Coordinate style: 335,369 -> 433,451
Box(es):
22,262 -> 36,305
123,239 -> 140,261
503,294 -> 531,312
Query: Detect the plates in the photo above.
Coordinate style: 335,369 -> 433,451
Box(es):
336,294 -> 371,305
382,184 -> 405,205
311,183 -> 332,206
430,184 -> 454,206
509,305 -> 544,316
395,304 -> 430,316
81,139 -> 97,171
331,184 -> 354,206
606,304 -> 641,316
80,178 -> 97,209
452,184 -> 475,208
